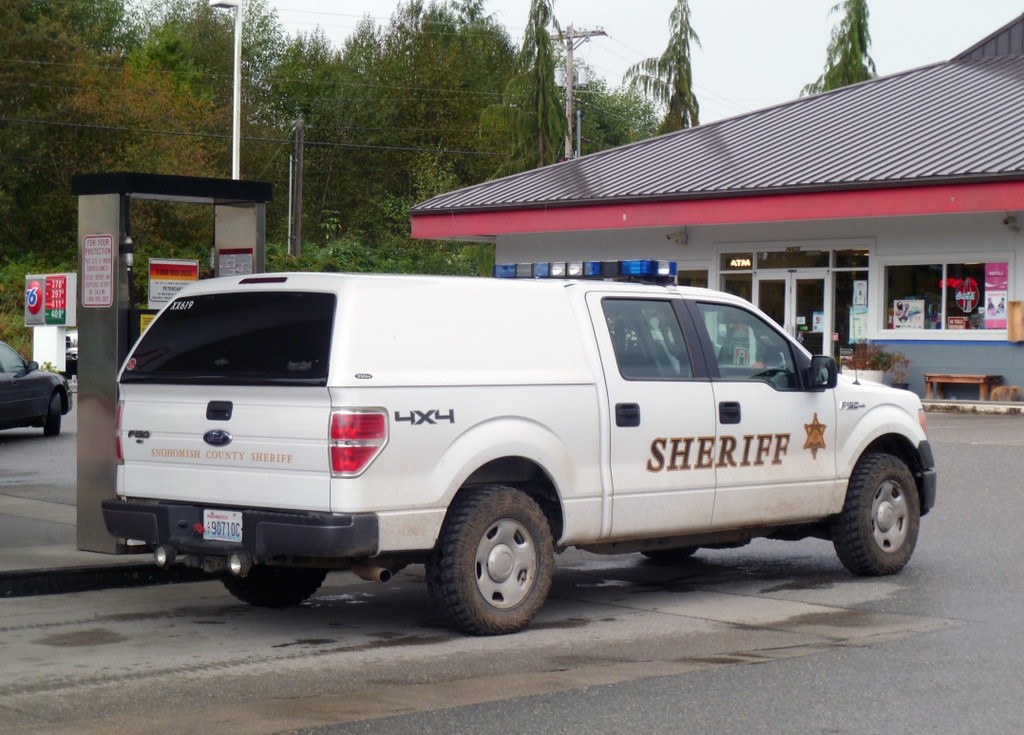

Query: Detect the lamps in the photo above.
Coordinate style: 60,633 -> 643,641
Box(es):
1005,217 -> 1016,228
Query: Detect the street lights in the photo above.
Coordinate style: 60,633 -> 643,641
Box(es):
208,0 -> 243,179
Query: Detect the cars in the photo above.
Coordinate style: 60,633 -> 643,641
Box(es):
0,341 -> 72,437
62,333 -> 78,379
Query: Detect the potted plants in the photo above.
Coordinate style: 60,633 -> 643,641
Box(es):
891,355 -> 910,390
841,337 -> 897,385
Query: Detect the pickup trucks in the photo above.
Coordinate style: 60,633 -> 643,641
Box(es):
102,273 -> 935,636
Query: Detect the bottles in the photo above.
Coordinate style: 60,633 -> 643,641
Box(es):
997,298 -> 1005,313
987,297 -> 995,315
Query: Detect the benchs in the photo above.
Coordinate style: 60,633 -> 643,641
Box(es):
923,373 -> 1003,401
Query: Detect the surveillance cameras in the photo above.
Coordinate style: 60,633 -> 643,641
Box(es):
665,231 -> 685,241
1003,215 -> 1016,228
